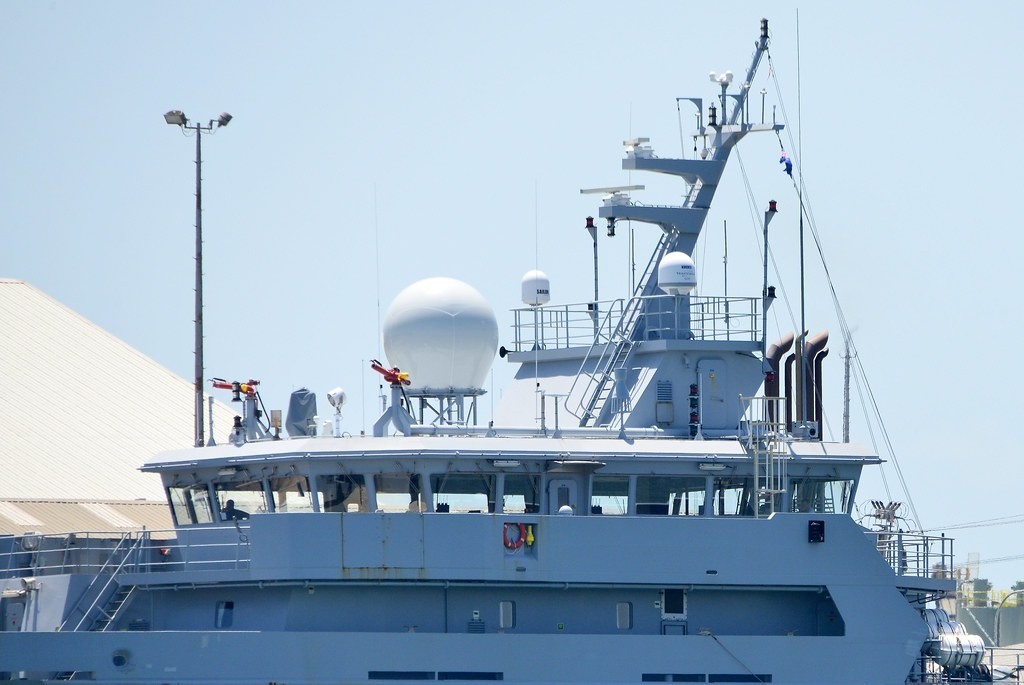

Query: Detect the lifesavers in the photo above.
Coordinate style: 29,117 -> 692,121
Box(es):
502,522 -> 528,550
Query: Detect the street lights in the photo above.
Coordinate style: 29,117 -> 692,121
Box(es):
162,109 -> 234,448
